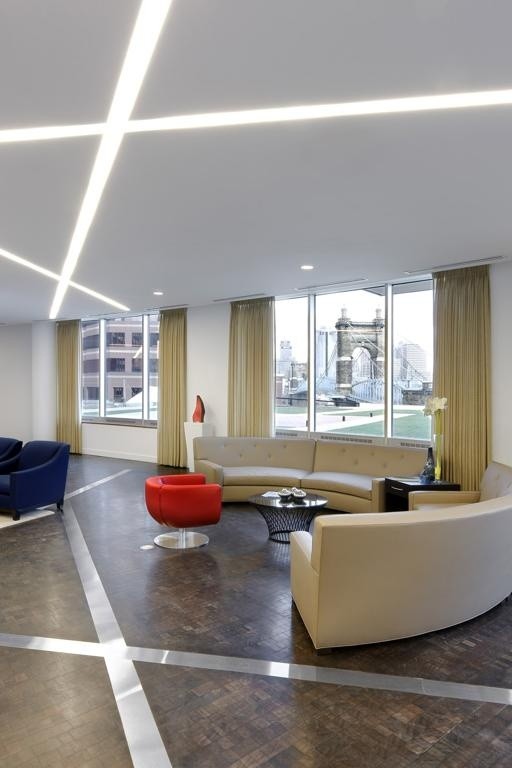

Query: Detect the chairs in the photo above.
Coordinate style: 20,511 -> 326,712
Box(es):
0,435 -> 71,523
144,473 -> 223,551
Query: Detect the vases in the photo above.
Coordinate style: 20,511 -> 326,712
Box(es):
431,411 -> 444,480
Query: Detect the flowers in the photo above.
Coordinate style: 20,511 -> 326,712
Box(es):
421,395 -> 448,416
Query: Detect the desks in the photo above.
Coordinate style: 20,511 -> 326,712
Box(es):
251,492 -> 327,544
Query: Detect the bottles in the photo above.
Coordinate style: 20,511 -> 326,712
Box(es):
424,447 -> 435,479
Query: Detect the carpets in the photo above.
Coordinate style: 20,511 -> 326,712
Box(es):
0,510 -> 55,529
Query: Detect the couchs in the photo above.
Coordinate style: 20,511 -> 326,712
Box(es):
289,459 -> 511,648
193,436 -> 431,515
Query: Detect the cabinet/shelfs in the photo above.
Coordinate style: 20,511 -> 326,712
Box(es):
382,477 -> 461,513
